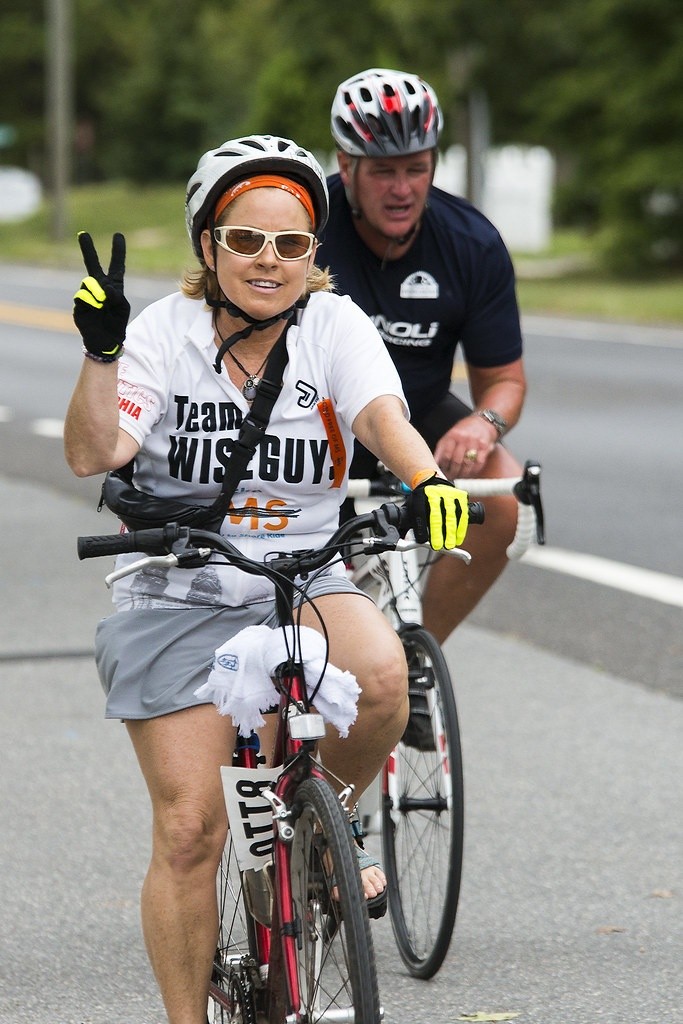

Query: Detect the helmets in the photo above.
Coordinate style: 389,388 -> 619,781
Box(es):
330,68 -> 444,157
185,135 -> 329,261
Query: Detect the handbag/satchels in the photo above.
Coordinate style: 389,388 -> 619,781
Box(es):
97,465 -> 228,568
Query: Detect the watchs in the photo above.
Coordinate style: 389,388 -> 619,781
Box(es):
472,407 -> 507,442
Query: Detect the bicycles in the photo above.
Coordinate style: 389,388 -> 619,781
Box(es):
309,458 -> 547,980
77,502 -> 488,1024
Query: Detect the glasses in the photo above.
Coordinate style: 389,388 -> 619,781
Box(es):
214,226 -> 315,261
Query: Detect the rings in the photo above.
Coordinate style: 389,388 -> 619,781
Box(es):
465,450 -> 478,461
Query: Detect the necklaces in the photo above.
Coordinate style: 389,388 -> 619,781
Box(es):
214,315 -> 270,404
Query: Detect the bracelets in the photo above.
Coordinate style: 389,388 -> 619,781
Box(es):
411,469 -> 439,489
81,344 -> 125,363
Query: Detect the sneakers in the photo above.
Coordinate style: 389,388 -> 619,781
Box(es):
403,644 -> 445,750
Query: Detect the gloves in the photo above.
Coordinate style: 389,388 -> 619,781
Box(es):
72,230 -> 131,357
407,469 -> 470,551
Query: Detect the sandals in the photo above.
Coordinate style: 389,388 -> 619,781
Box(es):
313,820 -> 387,909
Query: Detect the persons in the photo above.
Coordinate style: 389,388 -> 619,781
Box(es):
313,69 -> 530,753
62,134 -> 470,1024
